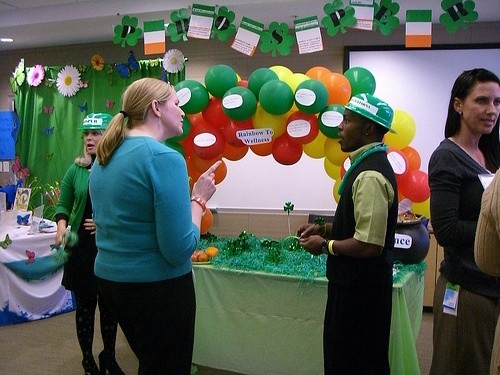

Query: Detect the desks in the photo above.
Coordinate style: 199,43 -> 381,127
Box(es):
0,214 -> 77,325
192,264 -> 423,375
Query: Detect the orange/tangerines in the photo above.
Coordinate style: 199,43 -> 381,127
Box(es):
199,247 -> 218,262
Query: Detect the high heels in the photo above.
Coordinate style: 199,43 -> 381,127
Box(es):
82,357 -> 101,374
98,349 -> 125,374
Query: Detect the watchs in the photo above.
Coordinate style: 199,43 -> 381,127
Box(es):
321,239 -> 329,254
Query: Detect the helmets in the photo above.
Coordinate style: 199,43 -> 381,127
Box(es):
77,113 -> 114,130
341,93 -> 397,135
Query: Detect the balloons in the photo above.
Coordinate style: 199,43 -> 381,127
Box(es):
165,115 -> 227,192
325,138 -> 349,203
175,65 -> 376,165
201,209 -> 214,233
383,111 -> 430,218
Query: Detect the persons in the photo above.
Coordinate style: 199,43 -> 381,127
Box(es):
297,93 -> 398,375
428,67 -> 500,375
474,168 -> 500,375
88,78 -> 222,375
55,113 -> 125,375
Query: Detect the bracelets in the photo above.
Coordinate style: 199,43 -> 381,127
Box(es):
315,222 -> 327,236
191,196 -> 206,215
328,239 -> 338,255
